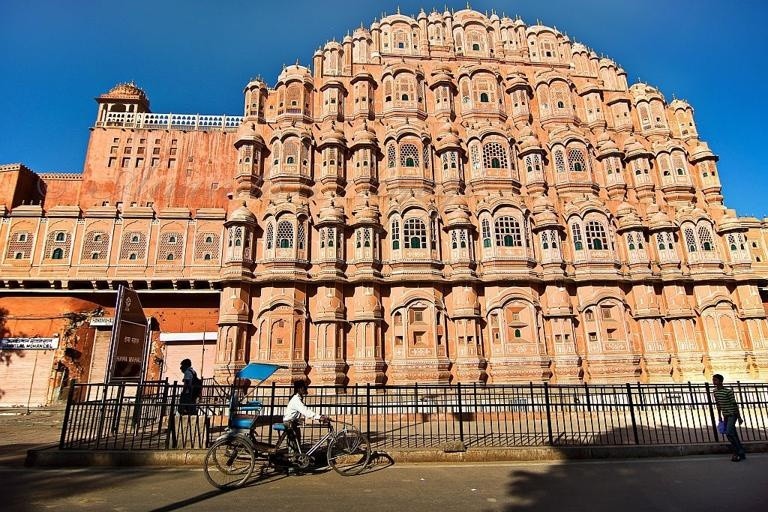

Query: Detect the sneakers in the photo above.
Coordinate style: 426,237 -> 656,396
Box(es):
730,453 -> 747,462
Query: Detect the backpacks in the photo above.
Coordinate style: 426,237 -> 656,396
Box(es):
187,368 -> 204,399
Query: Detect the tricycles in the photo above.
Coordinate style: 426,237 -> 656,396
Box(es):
203,362 -> 371,491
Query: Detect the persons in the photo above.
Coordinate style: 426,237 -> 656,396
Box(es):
174,358 -> 203,416
282,380 -> 330,474
712,374 -> 746,461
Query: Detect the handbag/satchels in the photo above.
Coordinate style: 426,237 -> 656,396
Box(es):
717,419 -> 726,434
